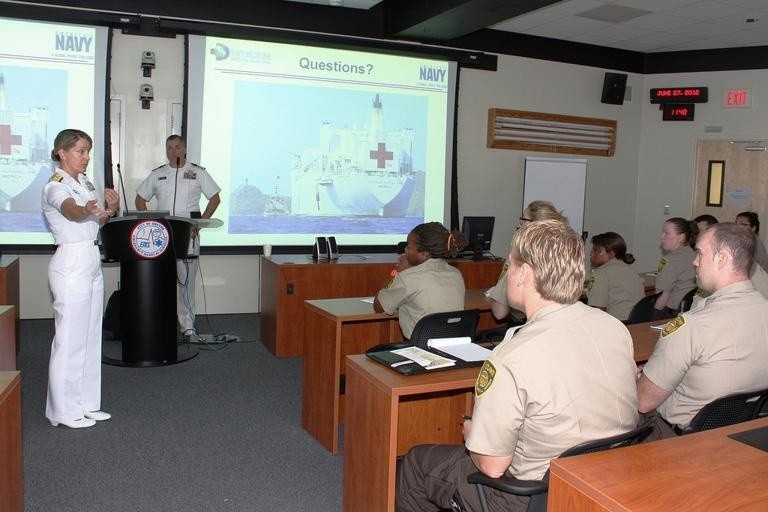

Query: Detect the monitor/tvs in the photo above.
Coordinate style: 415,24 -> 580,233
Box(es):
462,216 -> 495,261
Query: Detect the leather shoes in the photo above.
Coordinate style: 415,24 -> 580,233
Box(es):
183,329 -> 196,338
84,409 -> 112,422
50,417 -> 96,430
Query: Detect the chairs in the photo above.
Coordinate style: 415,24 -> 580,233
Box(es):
366,308 -> 480,355
468,421 -> 656,512
673,387 -> 768,435
666,285 -> 700,317
612,288 -> 664,323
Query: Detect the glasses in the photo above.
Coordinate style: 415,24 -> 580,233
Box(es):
518,217 -> 534,226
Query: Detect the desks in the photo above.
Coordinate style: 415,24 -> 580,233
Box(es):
541,414 -> 768,512
335,312 -> 684,512
1,252 -> 33,511
298,286 -> 514,457
260,248 -> 503,360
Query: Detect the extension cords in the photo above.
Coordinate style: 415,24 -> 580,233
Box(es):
190,333 -> 236,343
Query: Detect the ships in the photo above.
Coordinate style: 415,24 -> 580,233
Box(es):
261,185 -> 287,216
0,77 -> 53,217
286,93 -> 415,218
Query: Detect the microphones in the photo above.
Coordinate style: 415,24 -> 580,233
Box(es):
173,157 -> 180,217
117,164 -> 128,217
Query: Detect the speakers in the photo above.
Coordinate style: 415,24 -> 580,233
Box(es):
601,72 -> 628,105
327,236 -> 340,260
313,236 -> 328,260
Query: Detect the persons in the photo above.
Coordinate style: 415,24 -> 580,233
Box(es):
38,128 -> 121,432
586,231 -> 649,325
481,200 -> 572,321
693,211 -> 768,302
133,133 -> 223,341
371,219 -> 470,343
633,221 -> 768,445
653,216 -> 701,313
390,217 -> 642,511
